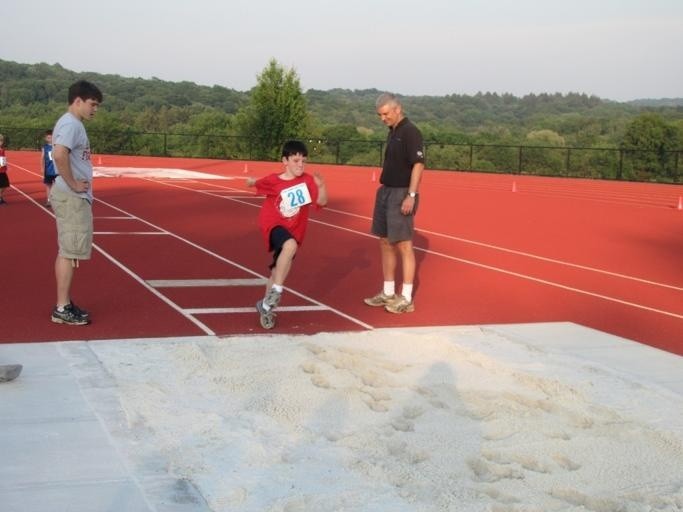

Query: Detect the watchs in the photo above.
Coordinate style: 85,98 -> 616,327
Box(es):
406,191 -> 420,198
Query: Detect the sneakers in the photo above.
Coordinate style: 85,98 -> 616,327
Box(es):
256,299 -> 274,329
364,291 -> 398,307
266,285 -> 281,308
51,305 -> 90,326
384,296 -> 414,314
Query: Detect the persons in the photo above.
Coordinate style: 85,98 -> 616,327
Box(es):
1,133 -> 10,204
247,140 -> 328,330
49,80 -> 104,326
364,93 -> 424,314
41,129 -> 59,208
